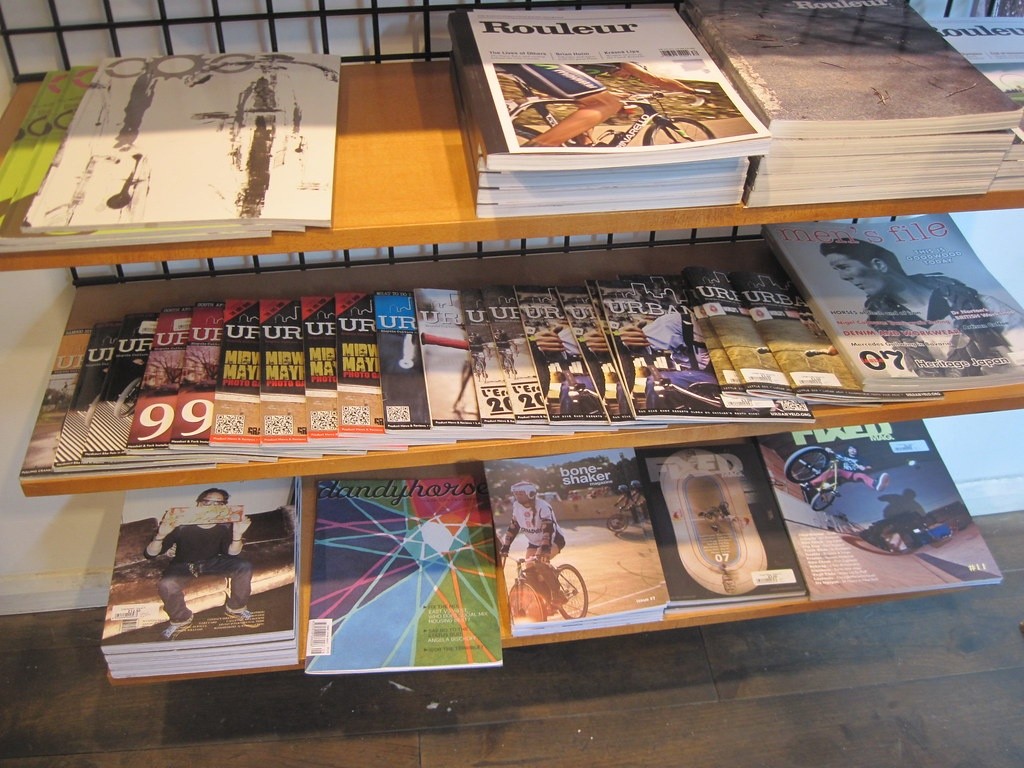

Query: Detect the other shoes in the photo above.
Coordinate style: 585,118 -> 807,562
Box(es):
550,590 -> 568,607
547,604 -> 558,615
873,473 -> 890,491
225,610 -> 260,628
800,483 -> 810,490
156,615 -> 194,641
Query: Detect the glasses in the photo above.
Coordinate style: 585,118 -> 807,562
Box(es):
201,499 -> 224,506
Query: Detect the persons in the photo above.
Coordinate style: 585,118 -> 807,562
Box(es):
493,330 -> 518,375
800,444 -> 890,492
503,62 -> 706,145
614,484 -> 635,509
624,480 -> 648,519
804,343 -> 839,357
616,304 -> 720,415
533,324 -> 597,419
146,487 -> 254,639
820,236 -> 1017,383
467,331 -> 493,378
577,326 -> 632,416
496,479 -> 568,617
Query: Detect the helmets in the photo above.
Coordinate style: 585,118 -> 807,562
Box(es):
619,485 -> 628,491
511,482 -> 537,499
631,480 -> 640,487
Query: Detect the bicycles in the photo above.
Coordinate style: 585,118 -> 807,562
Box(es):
471,350 -> 487,384
497,77 -> 716,148
499,350 -> 519,380
606,499 -> 647,533
783,444 -> 848,512
499,552 -> 589,625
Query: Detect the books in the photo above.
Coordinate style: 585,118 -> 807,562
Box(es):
446,0 -> 1024,221
100,419 -> 1004,690
19,214 -> 1024,480
0,50 -> 345,240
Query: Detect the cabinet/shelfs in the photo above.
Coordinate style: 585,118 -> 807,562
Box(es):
0,60 -> 1024,685
